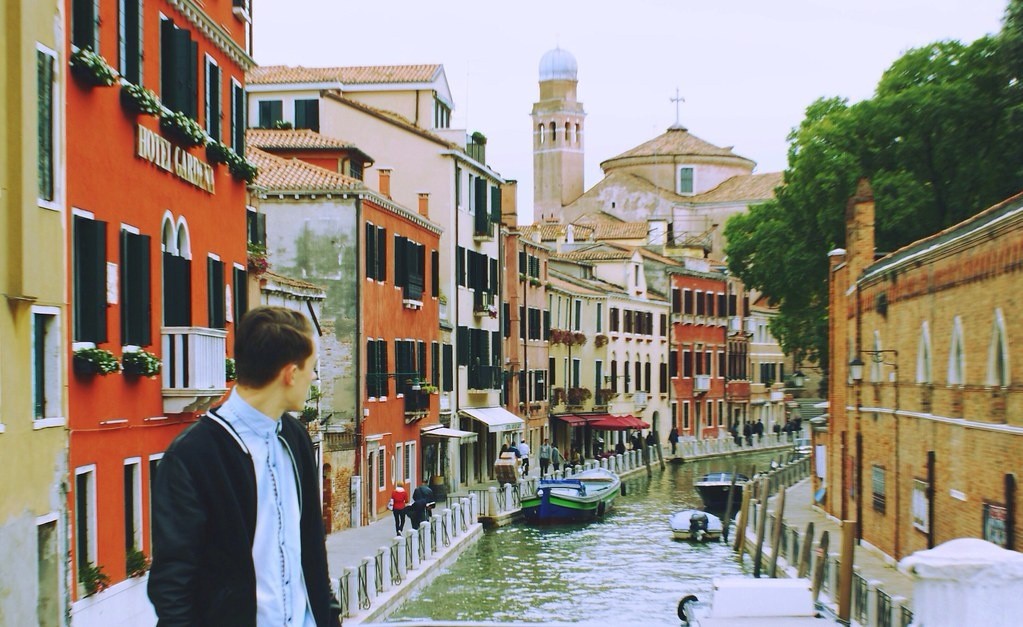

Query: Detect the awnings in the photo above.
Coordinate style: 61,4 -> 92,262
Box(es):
556,415 -> 586,428
463,407 -> 524,433
426,427 -> 479,444
579,414 -> 651,431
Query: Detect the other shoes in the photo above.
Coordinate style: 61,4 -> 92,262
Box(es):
398,529 -> 402,536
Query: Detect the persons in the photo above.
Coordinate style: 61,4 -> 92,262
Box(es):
646,431 -> 655,445
146,306 -> 344,627
615,440 -> 626,455
391,481 -> 409,536
630,433 -> 645,449
562,448 -> 584,478
730,421 -> 744,446
550,443 -> 565,471
507,441 -> 521,458
668,425 -> 680,454
499,444 -> 508,458
517,438 -> 530,478
744,419 -> 765,445
774,414 -> 803,442
539,438 -> 551,479
413,479 -> 434,504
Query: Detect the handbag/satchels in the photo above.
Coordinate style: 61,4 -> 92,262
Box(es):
387,498 -> 394,511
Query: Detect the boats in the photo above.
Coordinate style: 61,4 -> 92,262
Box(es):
670,509 -> 723,541
694,473 -> 749,511
520,467 -> 626,520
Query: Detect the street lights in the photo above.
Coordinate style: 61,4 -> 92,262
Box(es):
847,348 -> 900,572
